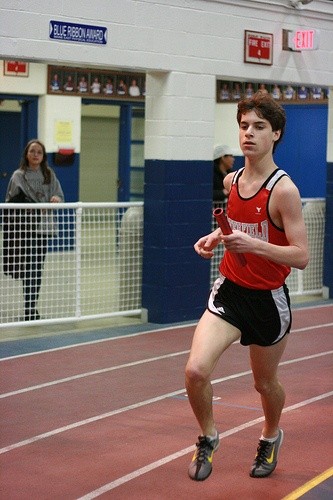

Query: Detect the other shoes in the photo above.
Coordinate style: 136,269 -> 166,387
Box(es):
25,308 -> 39,321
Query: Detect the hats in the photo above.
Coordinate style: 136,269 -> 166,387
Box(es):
214,144 -> 235,161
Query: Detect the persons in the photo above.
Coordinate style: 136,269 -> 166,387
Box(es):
217,82 -> 331,104
183,92 -> 309,481
49,73 -> 145,99
4,140 -> 65,322
213,145 -> 235,264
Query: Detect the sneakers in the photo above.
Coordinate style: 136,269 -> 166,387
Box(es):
249,429 -> 283,478
188,430 -> 220,481
